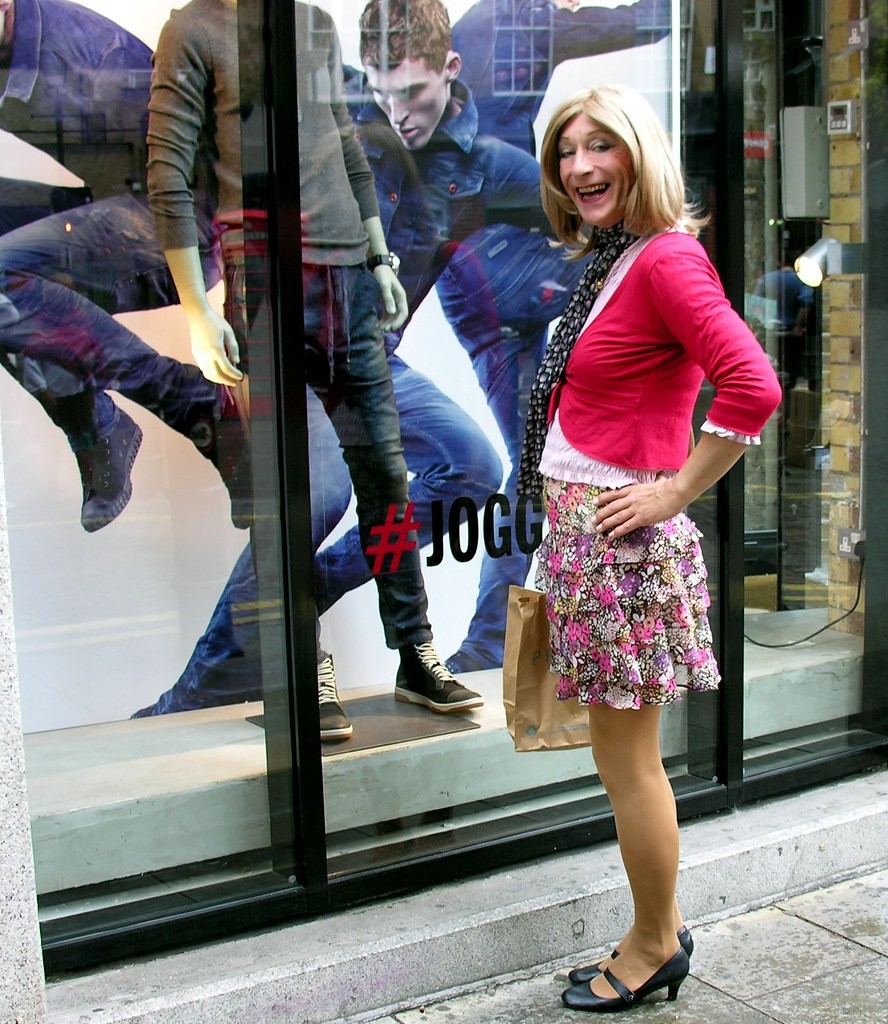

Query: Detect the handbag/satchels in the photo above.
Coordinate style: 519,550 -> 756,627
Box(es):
503,585 -> 593,753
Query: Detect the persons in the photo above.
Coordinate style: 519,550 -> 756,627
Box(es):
746,241 -> 811,482
412,1 -> 671,675
147,0 -> 487,738
1,1 -> 256,533
528,79 -> 783,1014
121,0 -> 589,718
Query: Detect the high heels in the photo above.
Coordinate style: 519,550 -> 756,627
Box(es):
563,944 -> 689,1013
568,925 -> 695,985
